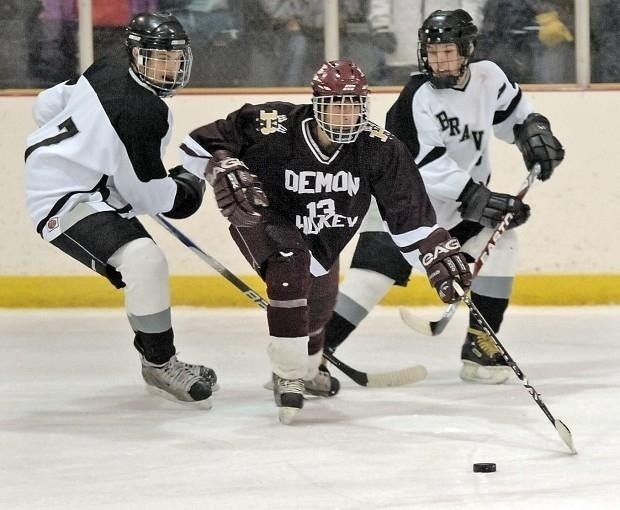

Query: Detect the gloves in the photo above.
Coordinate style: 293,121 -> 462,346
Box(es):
165,165 -> 208,220
419,226 -> 475,302
204,151 -> 272,229
514,111 -> 565,182
462,188 -> 532,228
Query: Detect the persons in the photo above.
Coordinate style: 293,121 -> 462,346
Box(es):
319,9 -> 565,376
2,2 -> 161,88
474,1 -> 619,82
25,12 -> 216,403
160,1 -> 483,87
178,60 -> 471,409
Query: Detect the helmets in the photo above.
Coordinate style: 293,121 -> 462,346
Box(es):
125,10 -> 188,60
416,10 -> 478,56
311,59 -> 369,96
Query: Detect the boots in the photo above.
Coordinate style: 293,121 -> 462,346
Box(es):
305,363 -> 340,396
273,373 -> 305,408
133,340 -> 216,403
459,329 -> 513,365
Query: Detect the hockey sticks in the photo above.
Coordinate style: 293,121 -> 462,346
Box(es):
452,280 -> 576,454
154,212 -> 426,387
400,162 -> 541,337
